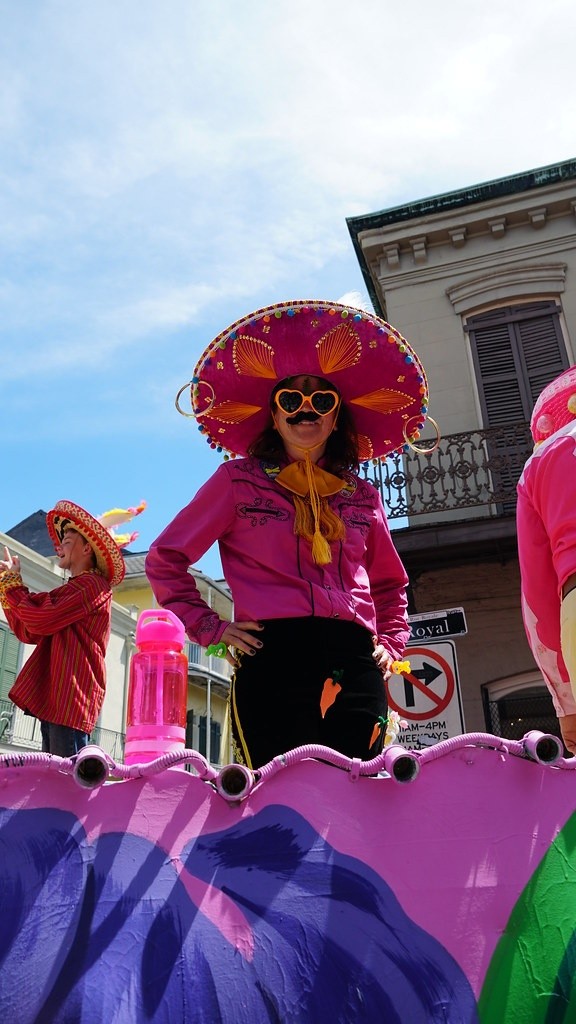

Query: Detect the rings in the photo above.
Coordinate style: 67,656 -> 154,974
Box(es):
205,642 -> 227,658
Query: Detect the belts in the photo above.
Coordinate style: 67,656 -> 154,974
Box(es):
559,573 -> 576,602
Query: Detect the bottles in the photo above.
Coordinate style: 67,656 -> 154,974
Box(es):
124,609 -> 189,767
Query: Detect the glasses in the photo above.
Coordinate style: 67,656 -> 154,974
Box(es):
274,388 -> 339,417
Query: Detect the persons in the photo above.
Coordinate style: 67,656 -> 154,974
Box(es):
144,299 -> 430,770
0,500 -> 147,759
517,363 -> 576,757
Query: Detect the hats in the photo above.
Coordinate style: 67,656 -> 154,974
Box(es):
46,499 -> 146,587
176,300 -> 441,470
